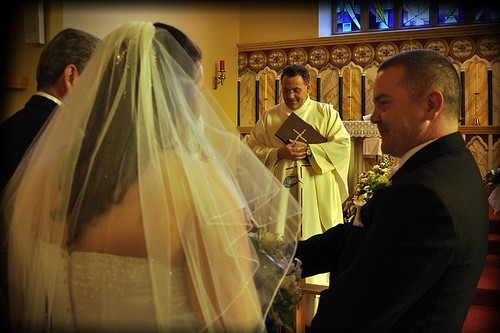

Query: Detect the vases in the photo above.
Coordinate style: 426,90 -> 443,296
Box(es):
354,205 -> 364,227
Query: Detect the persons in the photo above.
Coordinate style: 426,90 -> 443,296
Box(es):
0,28 -> 108,201
241,65 -> 352,243
287,50 -> 489,333
0,21 -> 267,333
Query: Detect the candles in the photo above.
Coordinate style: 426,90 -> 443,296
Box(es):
220,60 -> 224,71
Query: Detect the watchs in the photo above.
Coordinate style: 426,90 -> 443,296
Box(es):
306,144 -> 312,157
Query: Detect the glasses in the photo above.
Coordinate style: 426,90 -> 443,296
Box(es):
281,85 -> 306,93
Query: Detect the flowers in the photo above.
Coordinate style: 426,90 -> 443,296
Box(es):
246,222 -> 304,333
351,152 -> 400,208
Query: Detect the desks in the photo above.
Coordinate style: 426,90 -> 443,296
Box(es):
299,268 -> 330,327
